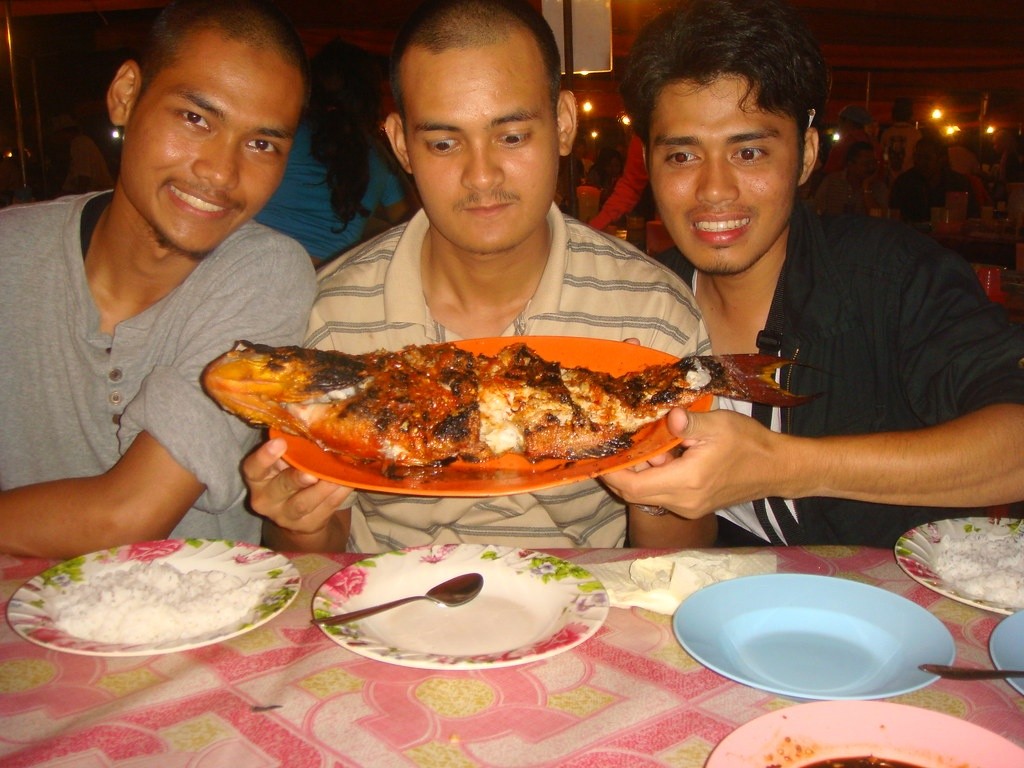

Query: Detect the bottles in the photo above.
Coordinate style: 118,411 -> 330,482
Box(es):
577,186 -> 601,219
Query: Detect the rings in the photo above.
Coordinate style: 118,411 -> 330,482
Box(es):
631,505 -> 667,519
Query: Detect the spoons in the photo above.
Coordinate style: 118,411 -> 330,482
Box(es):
312,573 -> 483,630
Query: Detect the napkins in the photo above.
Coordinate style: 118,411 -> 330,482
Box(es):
580,550 -> 780,617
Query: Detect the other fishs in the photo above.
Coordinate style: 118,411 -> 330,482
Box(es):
200,339 -> 825,469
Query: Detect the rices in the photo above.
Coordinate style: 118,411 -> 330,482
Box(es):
937,529 -> 1024,609
50,561 -> 268,648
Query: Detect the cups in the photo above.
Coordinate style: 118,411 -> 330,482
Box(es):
979,205 -> 994,221
978,266 -> 1000,298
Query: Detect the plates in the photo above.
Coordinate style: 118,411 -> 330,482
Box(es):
312,544 -> 609,669
700,701 -> 1024,768
894,516 -> 1024,615
670,572 -> 954,701
6,536 -> 302,659
986,611 -> 1024,698
269,336 -> 711,497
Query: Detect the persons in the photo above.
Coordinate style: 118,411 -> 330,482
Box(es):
561,138 -> 651,222
618,1 -> 1023,550
40,113 -> 114,198
246,36 -> 413,270
0,1 -> 317,560
803,97 -> 1024,267
238,1 -> 715,556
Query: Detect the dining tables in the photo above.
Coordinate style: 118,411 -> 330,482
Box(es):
0,543 -> 1024,768
920,217 -> 1024,244
